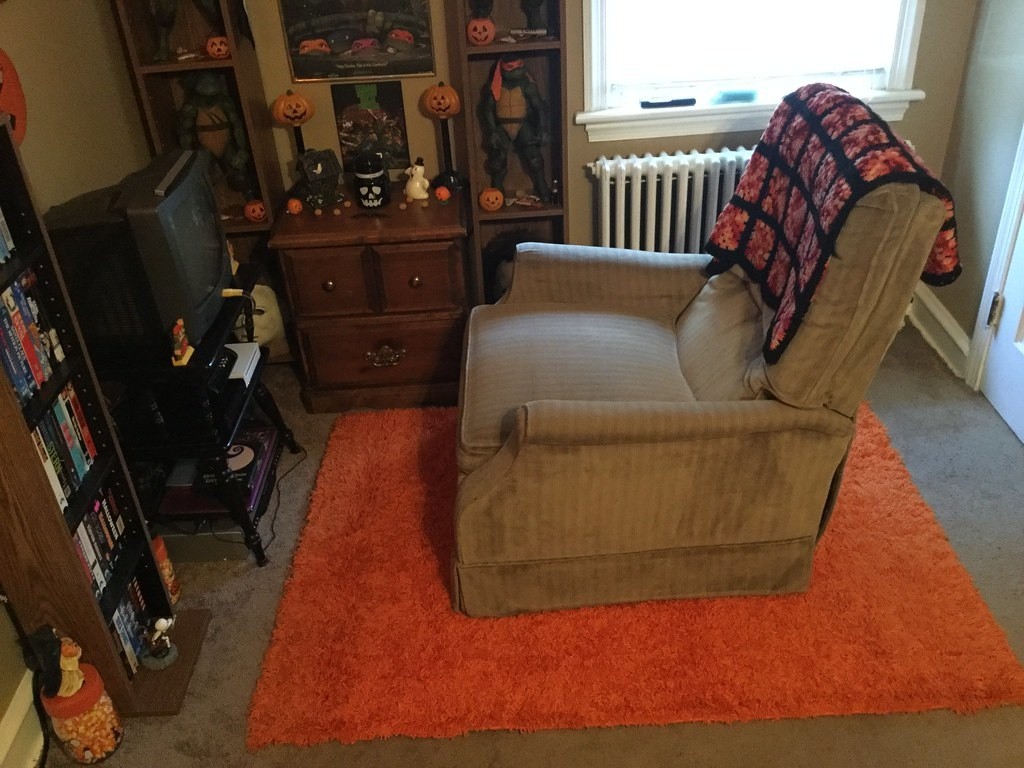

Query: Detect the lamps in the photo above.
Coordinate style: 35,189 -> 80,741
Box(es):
274,89 -> 316,198
421,80 -> 470,192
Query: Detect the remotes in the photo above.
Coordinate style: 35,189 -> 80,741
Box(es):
640,97 -> 696,108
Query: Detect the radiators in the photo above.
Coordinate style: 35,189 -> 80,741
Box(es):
586,143 -> 758,253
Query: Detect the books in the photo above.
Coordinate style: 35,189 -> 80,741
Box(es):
108,577 -> 152,678
0,264 -> 67,407
72,476 -> 134,602
0,203 -> 20,269
30,380 -> 99,516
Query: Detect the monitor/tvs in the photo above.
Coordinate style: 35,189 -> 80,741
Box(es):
41,148 -> 233,359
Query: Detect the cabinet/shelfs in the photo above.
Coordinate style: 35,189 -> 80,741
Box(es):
264,180 -> 473,412
116,0 -> 301,364
444,0 -> 571,303
0,113 -> 212,721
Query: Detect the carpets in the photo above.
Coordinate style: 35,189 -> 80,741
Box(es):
241,403 -> 1024,756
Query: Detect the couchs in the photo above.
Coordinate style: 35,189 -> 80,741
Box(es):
448,83 -> 950,619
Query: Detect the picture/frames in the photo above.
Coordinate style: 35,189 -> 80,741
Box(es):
276,0 -> 437,86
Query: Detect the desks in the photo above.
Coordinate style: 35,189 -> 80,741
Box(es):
89,229 -> 303,569
